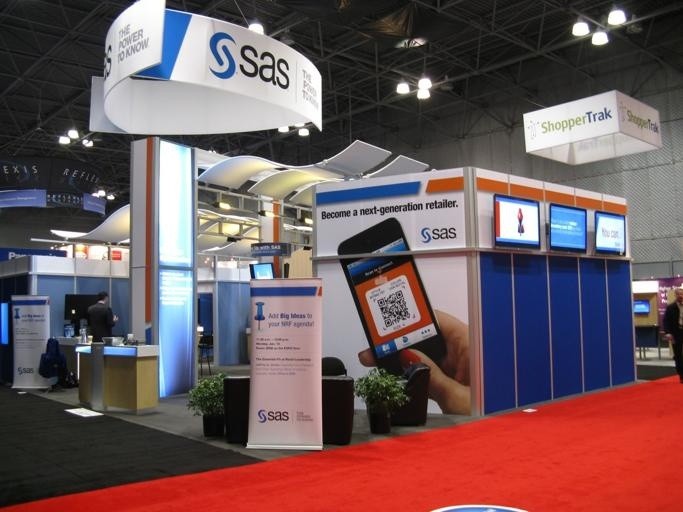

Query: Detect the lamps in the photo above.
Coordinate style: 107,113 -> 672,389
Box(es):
248,0 -> 264,34
395,37 -> 434,100
278,122 -> 310,138
91,188 -> 116,201
58,129 -> 94,148
570,0 -> 628,46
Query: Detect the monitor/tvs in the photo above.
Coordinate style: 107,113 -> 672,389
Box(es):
595,211 -> 625,256
550,203 -> 587,253
494,193 -> 540,249
634,300 -> 650,313
250,263 -> 275,279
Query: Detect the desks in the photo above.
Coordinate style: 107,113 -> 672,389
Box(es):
74,342 -> 160,416
222,377 -> 354,446
54,335 -> 94,386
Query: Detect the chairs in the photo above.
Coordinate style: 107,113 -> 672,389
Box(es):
197,330 -> 214,376
390,362 -> 431,427
323,357 -> 347,376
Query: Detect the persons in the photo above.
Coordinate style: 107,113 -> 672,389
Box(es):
353,302 -> 471,420
662,286 -> 683,387
86,291 -> 119,343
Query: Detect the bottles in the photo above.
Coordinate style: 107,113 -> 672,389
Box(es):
81,328 -> 86,343
127,334 -> 134,346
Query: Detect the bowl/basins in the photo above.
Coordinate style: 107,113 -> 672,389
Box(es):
101,337 -> 124,346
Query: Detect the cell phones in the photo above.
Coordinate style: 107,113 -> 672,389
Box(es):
338,218 -> 445,379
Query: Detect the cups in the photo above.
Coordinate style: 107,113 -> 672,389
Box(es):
86,335 -> 93,344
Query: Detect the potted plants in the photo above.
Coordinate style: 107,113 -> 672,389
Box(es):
352,366 -> 412,435
185,373 -> 228,438
